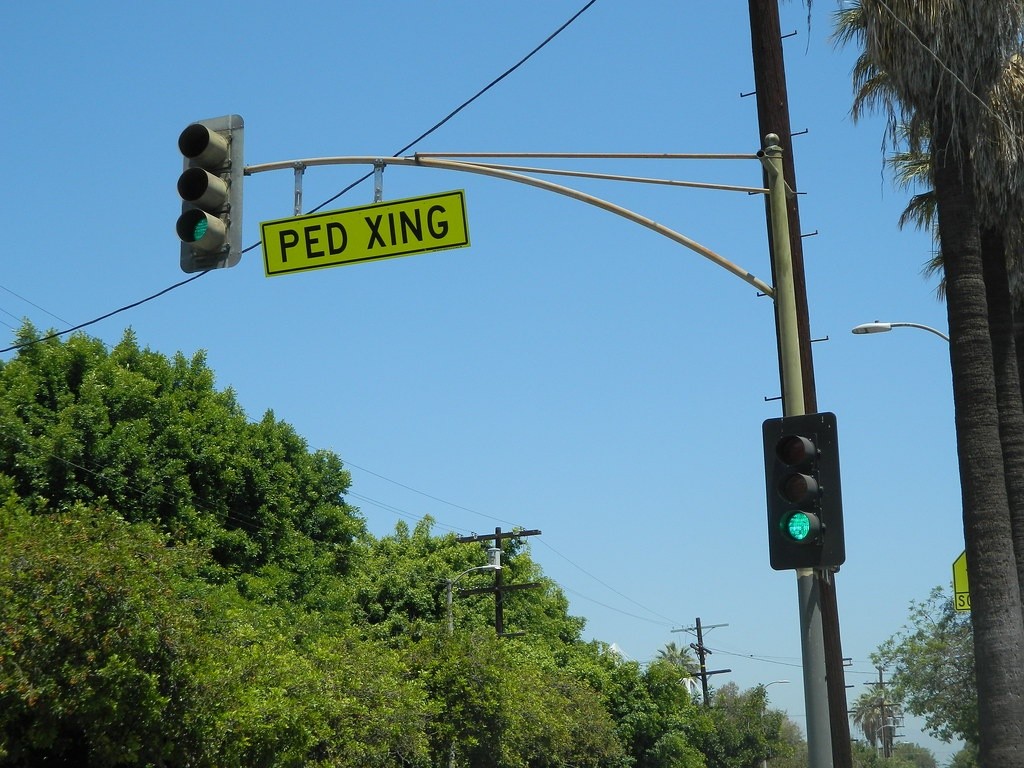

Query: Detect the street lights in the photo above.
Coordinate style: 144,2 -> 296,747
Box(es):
875,725 -> 895,759
447,563 -> 504,639
760,680 -> 790,767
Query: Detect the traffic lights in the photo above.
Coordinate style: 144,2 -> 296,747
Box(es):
762,412 -> 848,572
177,112 -> 248,271
